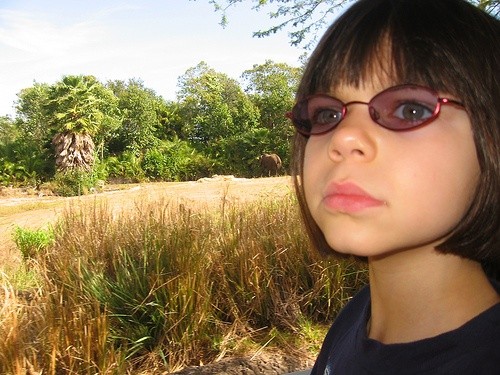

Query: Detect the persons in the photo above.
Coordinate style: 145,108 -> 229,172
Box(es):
288,1 -> 500,375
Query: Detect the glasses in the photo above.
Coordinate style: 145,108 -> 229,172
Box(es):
287,86 -> 465,132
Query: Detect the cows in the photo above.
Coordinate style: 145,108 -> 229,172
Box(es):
255,153 -> 282,177
